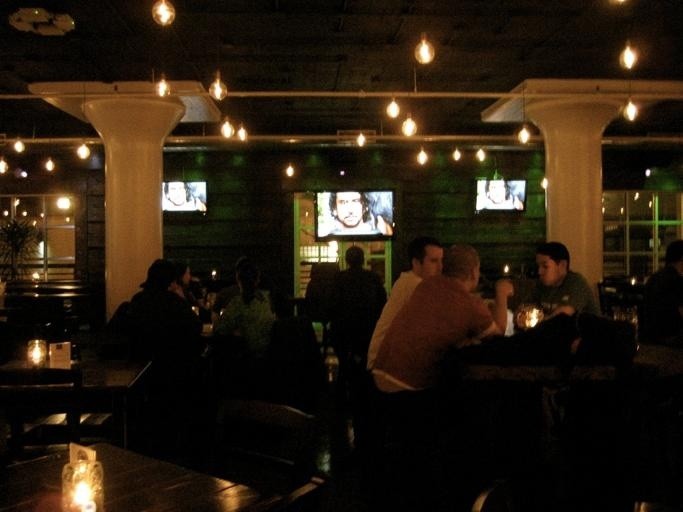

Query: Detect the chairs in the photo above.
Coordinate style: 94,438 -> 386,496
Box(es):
0,362 -> 83,450
0,385 -> 137,462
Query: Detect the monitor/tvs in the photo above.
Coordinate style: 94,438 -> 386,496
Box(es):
315,189 -> 396,242
475,178 -> 528,213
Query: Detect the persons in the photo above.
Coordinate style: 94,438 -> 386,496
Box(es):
476,179 -> 524,210
318,191 -> 393,235
75,235 -> 683,441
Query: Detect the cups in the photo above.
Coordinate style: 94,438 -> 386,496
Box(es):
27,339 -> 48,370
524,307 -> 545,330
514,304 -> 534,328
59,459 -> 106,512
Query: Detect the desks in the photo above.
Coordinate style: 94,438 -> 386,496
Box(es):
0,441 -> 262,512
0,358 -> 153,449
